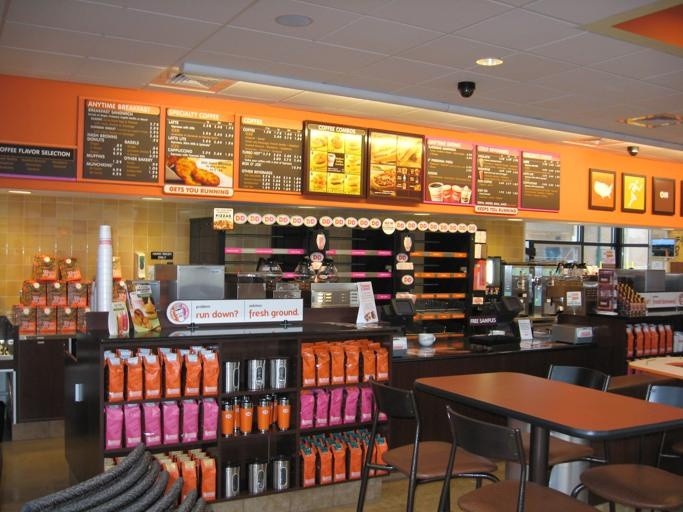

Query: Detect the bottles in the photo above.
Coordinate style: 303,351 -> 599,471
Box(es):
221,393 -> 291,438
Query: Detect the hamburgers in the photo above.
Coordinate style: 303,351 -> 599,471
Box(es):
311,134 -> 359,194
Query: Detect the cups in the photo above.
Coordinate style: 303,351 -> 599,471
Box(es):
428,182 -> 472,204
419,333 -> 436,346
97,225 -> 113,336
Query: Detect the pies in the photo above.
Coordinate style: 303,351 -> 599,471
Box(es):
371,172 -> 396,192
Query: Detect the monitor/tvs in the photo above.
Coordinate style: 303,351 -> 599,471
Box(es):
502,295 -> 524,311
564,289 -> 585,311
392,298 -> 416,316
650,238 -> 675,258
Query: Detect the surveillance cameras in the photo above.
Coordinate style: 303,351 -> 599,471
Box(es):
458,82 -> 475,97
627,146 -> 639,156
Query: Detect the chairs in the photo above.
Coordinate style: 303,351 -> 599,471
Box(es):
521,365 -> 610,486
356,376 -> 498,512
438,407 -> 598,510
573,385 -> 681,512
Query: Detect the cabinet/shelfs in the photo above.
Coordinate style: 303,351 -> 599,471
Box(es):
63,335 -> 392,502
0,315 -> 91,423
557,308 -> 682,374
482,258 -> 674,316
391,330 -> 608,437
187,214 -> 475,330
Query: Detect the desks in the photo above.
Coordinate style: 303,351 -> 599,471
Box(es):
417,372 -> 680,484
